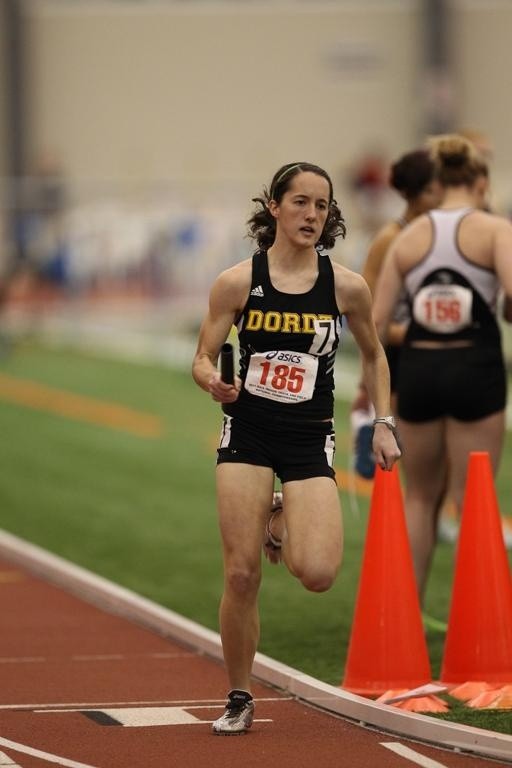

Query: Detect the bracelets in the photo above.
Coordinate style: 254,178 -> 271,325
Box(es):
373,415 -> 396,429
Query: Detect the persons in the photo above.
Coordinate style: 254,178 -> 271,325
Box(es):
367,131 -> 512,625
187,158 -> 407,737
352,141 -> 459,543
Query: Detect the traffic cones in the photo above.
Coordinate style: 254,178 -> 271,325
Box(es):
342,460 -> 435,697
439,451 -> 511,690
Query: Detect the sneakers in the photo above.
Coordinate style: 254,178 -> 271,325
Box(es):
265,490 -> 283,565
212,690 -> 255,736
